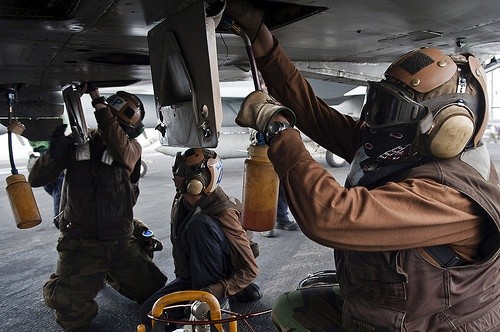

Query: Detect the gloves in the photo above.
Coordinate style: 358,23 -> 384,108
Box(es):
84,81 -> 98,93
50,123 -> 75,156
236,90 -> 296,147
207,0 -> 264,45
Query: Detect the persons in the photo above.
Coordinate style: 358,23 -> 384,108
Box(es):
260,179 -> 298,238
222,0 -> 500,332
28,90 -> 168,332
137,147 -> 262,324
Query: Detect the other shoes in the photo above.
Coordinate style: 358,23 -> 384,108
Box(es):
234,283 -> 264,301
276,221 -> 297,231
263,230 -> 275,237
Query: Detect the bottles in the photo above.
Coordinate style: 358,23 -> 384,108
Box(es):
5,169 -> 42,229
240,131 -> 279,231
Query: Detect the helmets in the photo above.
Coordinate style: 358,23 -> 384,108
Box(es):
182,147 -> 223,196
106,91 -> 145,138
384,47 -> 489,158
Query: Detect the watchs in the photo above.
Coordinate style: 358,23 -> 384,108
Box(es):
265,121 -> 292,147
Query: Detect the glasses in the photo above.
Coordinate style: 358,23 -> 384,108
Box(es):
361,79 -> 420,128
173,152 -> 190,176
